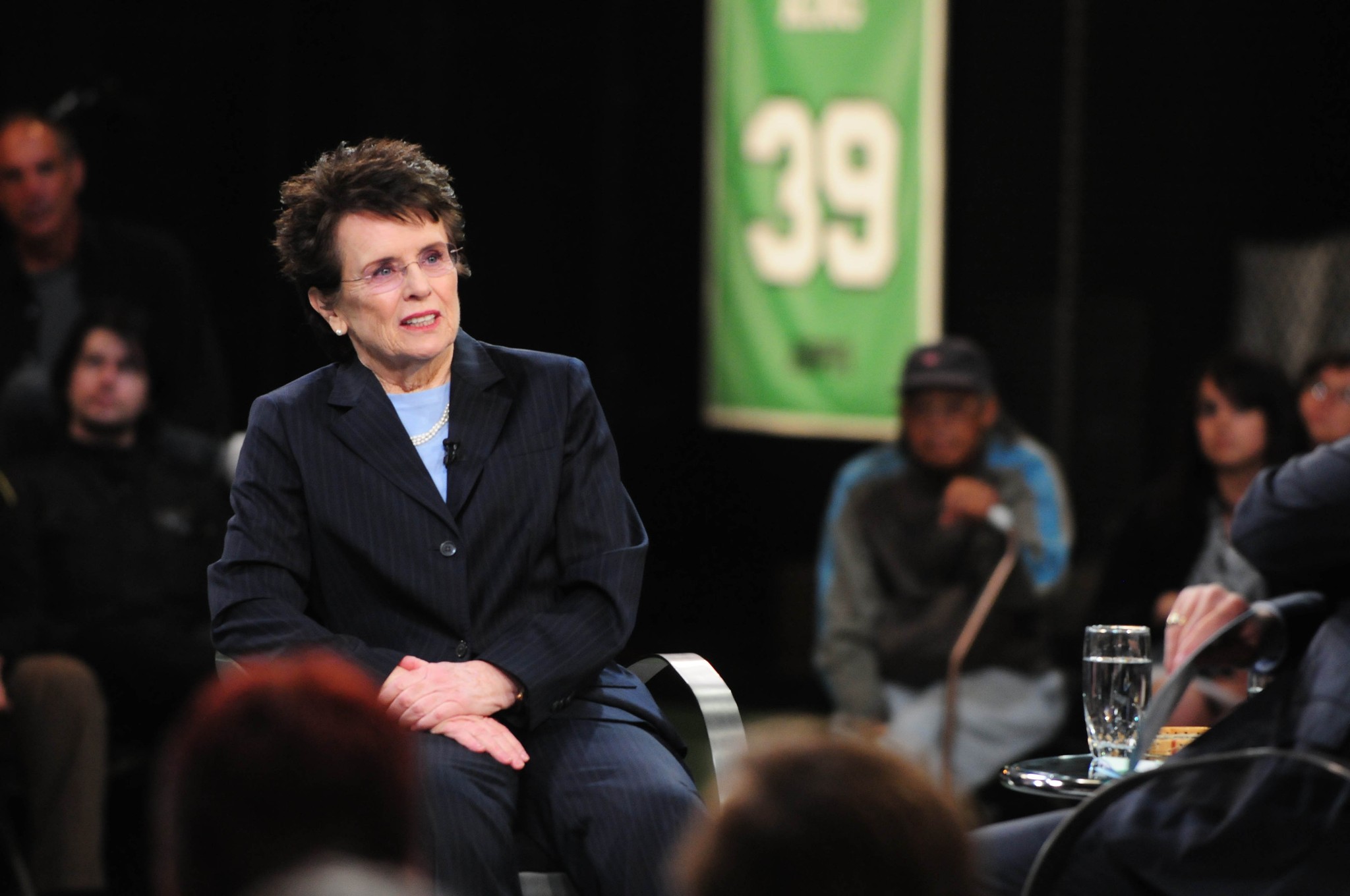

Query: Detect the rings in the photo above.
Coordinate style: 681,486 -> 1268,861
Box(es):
1166,613 -> 1184,626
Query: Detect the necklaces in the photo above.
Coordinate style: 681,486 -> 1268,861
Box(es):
410,404 -> 449,444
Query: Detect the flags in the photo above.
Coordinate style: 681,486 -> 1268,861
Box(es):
707,0 -> 942,441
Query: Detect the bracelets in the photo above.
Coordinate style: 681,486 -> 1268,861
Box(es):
517,688 -> 524,700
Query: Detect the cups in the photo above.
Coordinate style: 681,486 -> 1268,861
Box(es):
1083,624 -> 1153,781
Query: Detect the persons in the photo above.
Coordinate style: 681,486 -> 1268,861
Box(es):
956,438 -> 1350,896
0,121 -> 417,896
813,339 -> 1075,799
1278,351 -> 1350,456
1145,350 -> 1288,726
676,716 -> 971,896
207,141 -> 708,896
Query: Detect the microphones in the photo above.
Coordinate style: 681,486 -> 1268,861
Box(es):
443,435 -> 461,465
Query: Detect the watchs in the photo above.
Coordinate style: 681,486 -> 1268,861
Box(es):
986,503 -> 1016,533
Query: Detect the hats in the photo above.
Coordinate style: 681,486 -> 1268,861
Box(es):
903,339 -> 988,389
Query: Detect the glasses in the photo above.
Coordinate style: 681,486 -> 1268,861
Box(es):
345,242 -> 466,296
905,393 -> 979,417
1196,394 -> 1258,420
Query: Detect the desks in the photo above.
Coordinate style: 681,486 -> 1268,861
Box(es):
997,754 -> 1100,800
